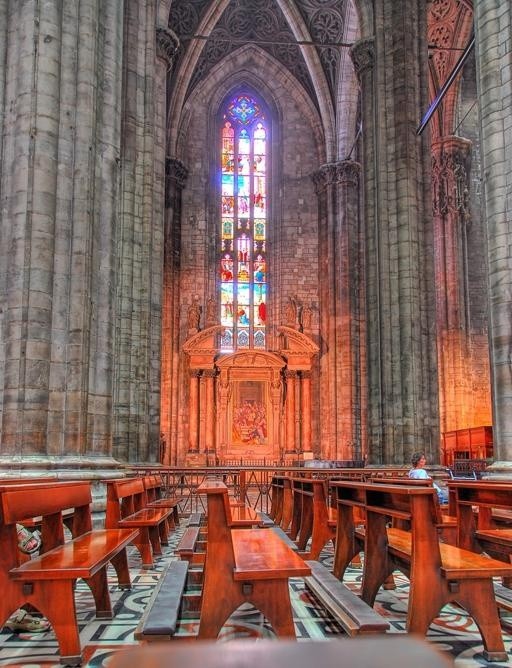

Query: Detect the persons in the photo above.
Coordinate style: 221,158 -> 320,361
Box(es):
408,451 -> 447,505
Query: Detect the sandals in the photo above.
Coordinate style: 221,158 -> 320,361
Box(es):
0,610 -> 51,634
16,523 -> 42,554
